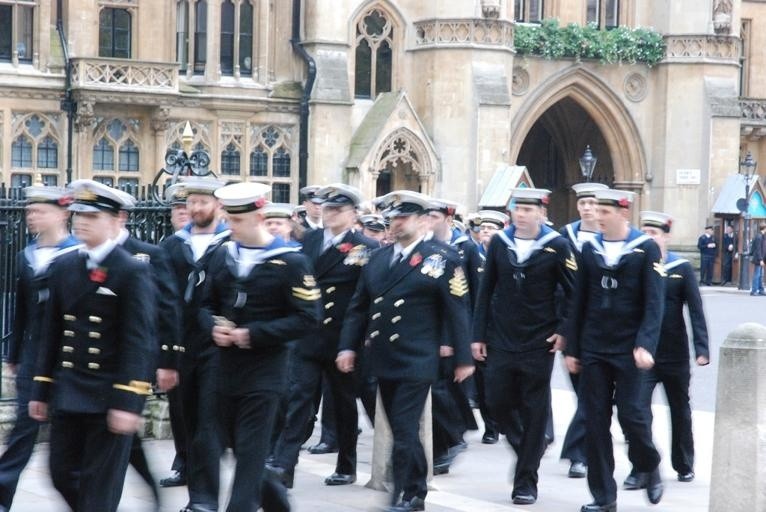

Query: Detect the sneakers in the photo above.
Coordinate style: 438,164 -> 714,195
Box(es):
750,290 -> 765,297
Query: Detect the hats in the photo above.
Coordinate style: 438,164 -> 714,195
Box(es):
638,212 -> 673,232
298,186 -> 457,229
509,188 -> 552,205
467,210 -> 510,234
571,183 -> 634,208
164,176 -> 292,217
21,179 -> 137,215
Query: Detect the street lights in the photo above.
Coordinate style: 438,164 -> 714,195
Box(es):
738,148 -> 759,291
578,144 -> 598,182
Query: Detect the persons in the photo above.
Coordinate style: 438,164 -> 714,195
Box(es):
750,223 -> 766,296
719,224 -> 739,287
0,175 -> 714,511
696,225 -> 719,286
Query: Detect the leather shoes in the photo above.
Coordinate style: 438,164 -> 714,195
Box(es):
581,502 -> 616,512
483,433 -> 497,444
393,496 -> 424,512
700,280 -> 726,286
324,472 -> 357,485
678,472 -> 694,481
513,495 -> 535,504
624,469 -> 661,505
569,461 -> 586,478
159,472 -> 188,486
309,440 -> 338,453
